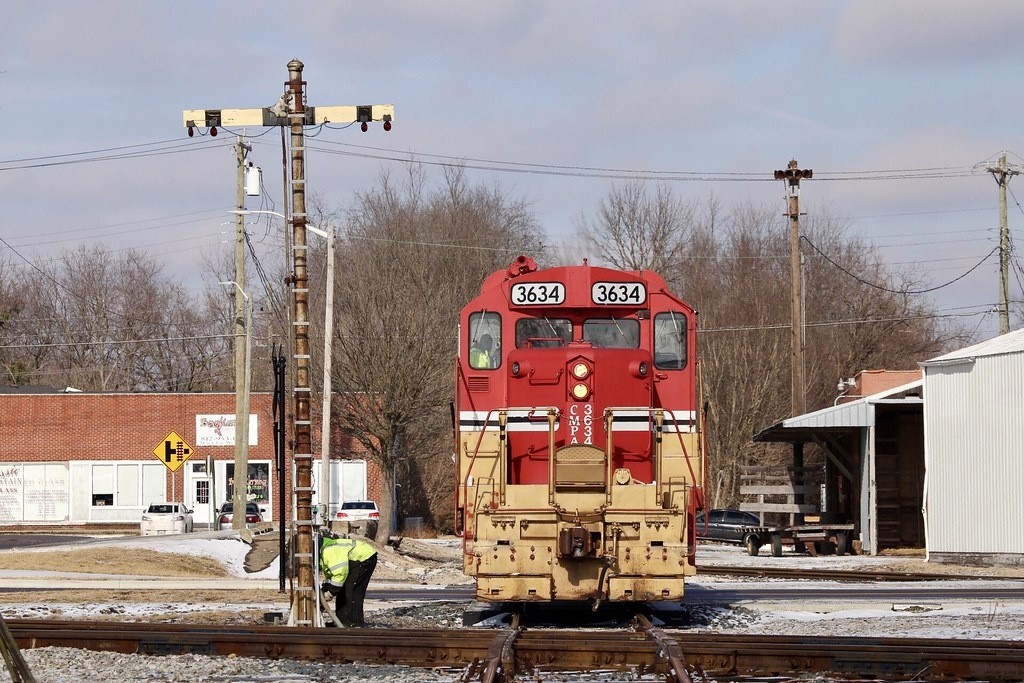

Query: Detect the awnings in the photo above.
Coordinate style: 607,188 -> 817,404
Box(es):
752,401 -> 867,482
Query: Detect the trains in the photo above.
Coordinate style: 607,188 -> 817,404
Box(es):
449,256 -> 709,603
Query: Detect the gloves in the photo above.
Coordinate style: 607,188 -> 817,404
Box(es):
323,590 -> 334,601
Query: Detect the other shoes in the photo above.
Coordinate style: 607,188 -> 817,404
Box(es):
325,621 -> 338,627
354,624 -> 362,628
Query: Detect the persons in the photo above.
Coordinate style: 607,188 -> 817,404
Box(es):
313,533 -> 378,628
469,334 -> 493,369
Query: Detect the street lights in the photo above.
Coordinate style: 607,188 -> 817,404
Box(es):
218,280 -> 253,529
228,209 -> 336,525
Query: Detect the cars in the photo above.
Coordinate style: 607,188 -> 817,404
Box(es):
695,508 -> 780,546
213,501 -> 266,529
140,501 -> 194,535
333,500 -> 379,521
